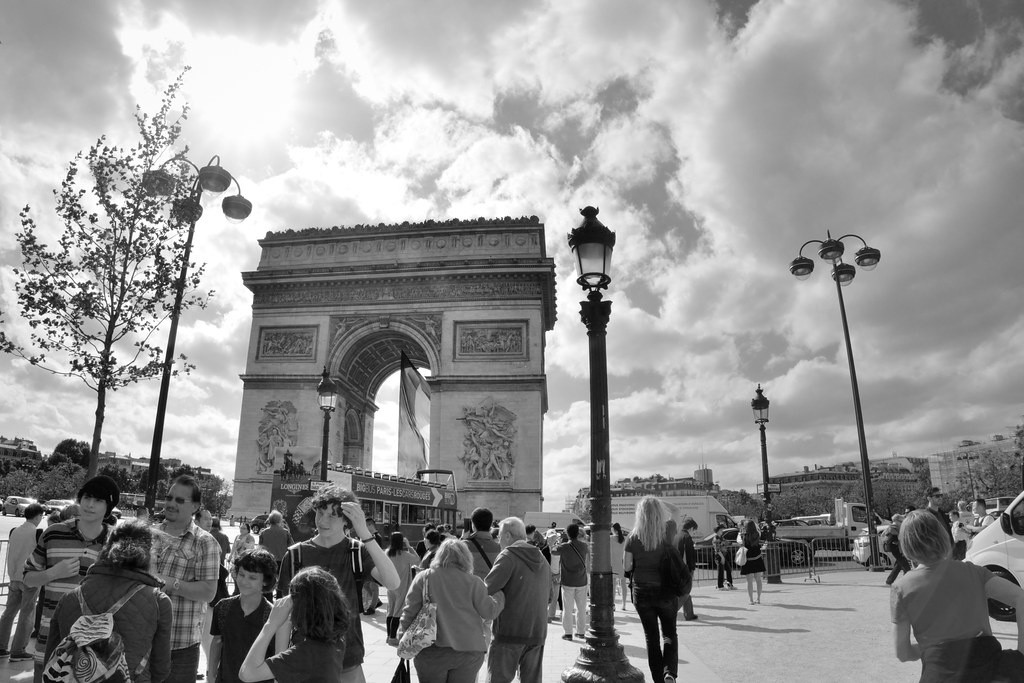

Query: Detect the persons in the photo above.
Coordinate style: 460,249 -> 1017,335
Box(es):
456,400 -> 518,480
461,328 -> 522,353
890,509 -> 1024,683
255,278 -> 542,302
415,315 -> 441,345
761,525 -> 777,542
330,318 -> 361,347
886,486 -> 995,588
284,448 -> 306,474
0,475 -> 698,683
855,509 -> 867,522
256,400 -> 300,472
264,331 -> 313,354
712,525 -> 737,591
740,520 -> 767,605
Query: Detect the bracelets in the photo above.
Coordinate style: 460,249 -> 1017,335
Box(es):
362,535 -> 375,543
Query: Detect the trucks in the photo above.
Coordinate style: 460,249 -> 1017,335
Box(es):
524,511 -> 589,529
764,494 -> 892,566
609,496 -> 738,545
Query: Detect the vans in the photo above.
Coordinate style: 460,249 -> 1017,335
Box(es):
959,491 -> 1024,622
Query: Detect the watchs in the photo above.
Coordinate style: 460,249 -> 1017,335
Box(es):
172,578 -> 181,596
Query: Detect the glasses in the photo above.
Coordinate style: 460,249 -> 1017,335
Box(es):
931,494 -> 943,498
165,494 -> 195,505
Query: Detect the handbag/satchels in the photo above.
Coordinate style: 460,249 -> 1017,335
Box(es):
397,568 -> 437,660
660,541 -> 692,596
734,546 -> 748,565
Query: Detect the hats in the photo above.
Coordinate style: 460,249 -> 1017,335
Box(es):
546,529 -> 557,537
892,513 -> 906,521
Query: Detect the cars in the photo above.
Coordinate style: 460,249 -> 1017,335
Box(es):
689,527 -> 811,569
2,496 -> 32,517
41,500 -> 74,516
112,507 -> 122,519
851,525 -> 897,569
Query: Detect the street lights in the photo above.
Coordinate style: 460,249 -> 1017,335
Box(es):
790,228 -> 885,575
561,204 -> 646,683
139,150 -> 256,516
317,367 -> 338,486
750,382 -> 786,587
956,451 -> 976,498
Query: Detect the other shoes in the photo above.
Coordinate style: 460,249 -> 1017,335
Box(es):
575,633 -> 584,638
563,634 -> 572,640
728,586 -> 738,590
664,673 -> 676,683
754,599 -> 761,603
376,601 -> 383,607
386,636 -> 399,645
9,651 -> 35,661
749,602 -> 754,605
685,615 -> 697,620
363,607 -> 375,614
717,587 -> 728,591
0,650 -> 10,658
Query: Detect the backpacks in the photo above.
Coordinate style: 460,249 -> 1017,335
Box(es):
41,583 -> 152,683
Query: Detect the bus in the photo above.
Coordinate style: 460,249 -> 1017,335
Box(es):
981,496 -> 1017,517
309,460 -> 463,549
